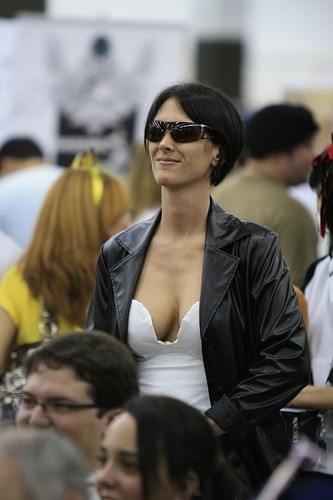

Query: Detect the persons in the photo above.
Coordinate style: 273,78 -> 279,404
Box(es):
0,103 -> 333,500
17,328 -> 141,500
0,426 -> 94,500
96,395 -> 246,500
90,84 -> 312,499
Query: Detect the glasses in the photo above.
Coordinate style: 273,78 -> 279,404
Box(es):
10,394 -> 99,418
144,119 -> 213,143
